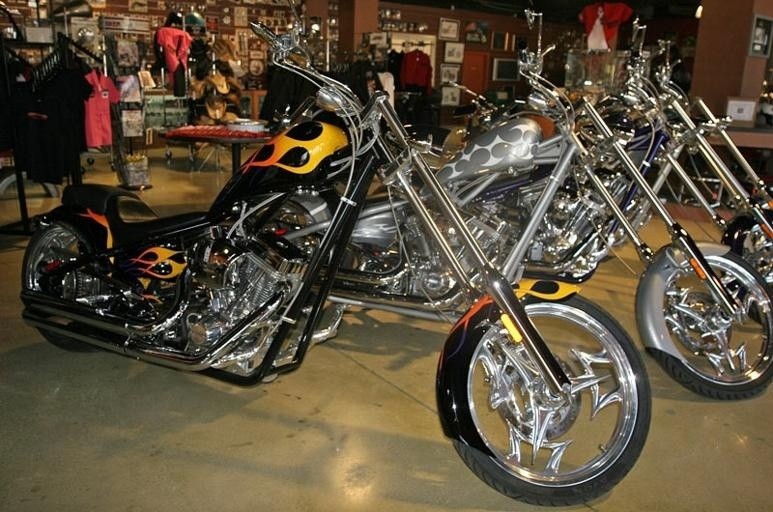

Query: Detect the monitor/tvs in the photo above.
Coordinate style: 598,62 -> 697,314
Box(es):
492,58 -> 520,81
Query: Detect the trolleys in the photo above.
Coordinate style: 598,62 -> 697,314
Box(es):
156,30 -> 221,169
71,44 -> 127,176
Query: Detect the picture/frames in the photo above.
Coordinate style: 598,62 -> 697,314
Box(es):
436,16 -> 530,112
747,13 -> 772,60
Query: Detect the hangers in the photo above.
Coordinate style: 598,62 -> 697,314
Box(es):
5,45 -> 104,86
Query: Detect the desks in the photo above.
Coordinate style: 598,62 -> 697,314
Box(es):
159,127 -> 273,185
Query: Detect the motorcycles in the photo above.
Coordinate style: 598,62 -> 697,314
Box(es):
20,0 -> 653,509
222,2 -> 773,401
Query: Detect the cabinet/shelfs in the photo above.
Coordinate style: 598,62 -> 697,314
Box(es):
241,91 -> 267,122
